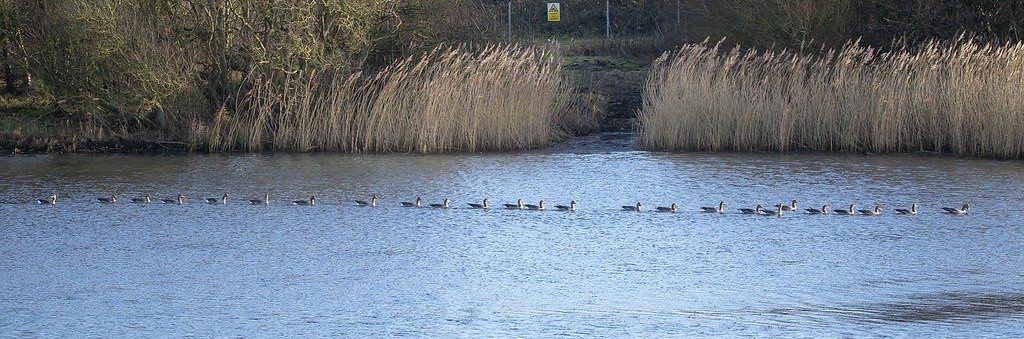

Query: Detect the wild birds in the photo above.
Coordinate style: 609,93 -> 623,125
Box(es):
97,189 -> 378,208
398,191 -> 578,215
622,199 -> 971,219
37,193 -> 58,206
